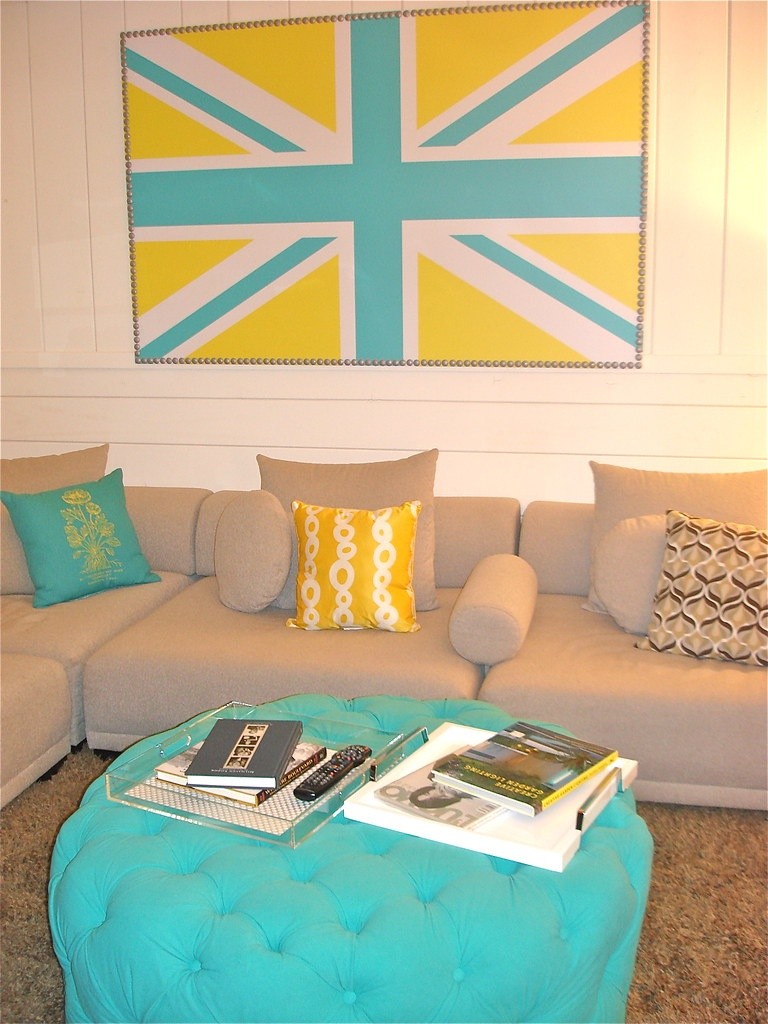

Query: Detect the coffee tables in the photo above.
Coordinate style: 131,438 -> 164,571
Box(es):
47,693 -> 654,1024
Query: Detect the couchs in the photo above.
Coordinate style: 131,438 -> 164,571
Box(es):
0,488 -> 768,811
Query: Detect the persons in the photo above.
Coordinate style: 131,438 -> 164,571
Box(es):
408,785 -> 473,808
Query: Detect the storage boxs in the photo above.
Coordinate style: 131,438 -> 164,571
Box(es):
105,701 -> 404,849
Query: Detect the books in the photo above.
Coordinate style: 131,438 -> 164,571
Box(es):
156,738 -> 327,807
185,718 -> 302,790
373,744 -> 509,833
344,722 -> 636,873
429,721 -> 618,817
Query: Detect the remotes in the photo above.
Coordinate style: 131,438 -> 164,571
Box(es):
294,745 -> 373,801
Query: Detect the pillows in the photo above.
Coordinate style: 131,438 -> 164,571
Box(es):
0,466 -> 161,608
0,443 -> 109,595
256,448 -> 441,612
631,510 -> 768,667
591,515 -> 670,636
448,553 -> 537,665
582,460 -> 768,615
286,499 -> 422,633
214,489 -> 293,612
